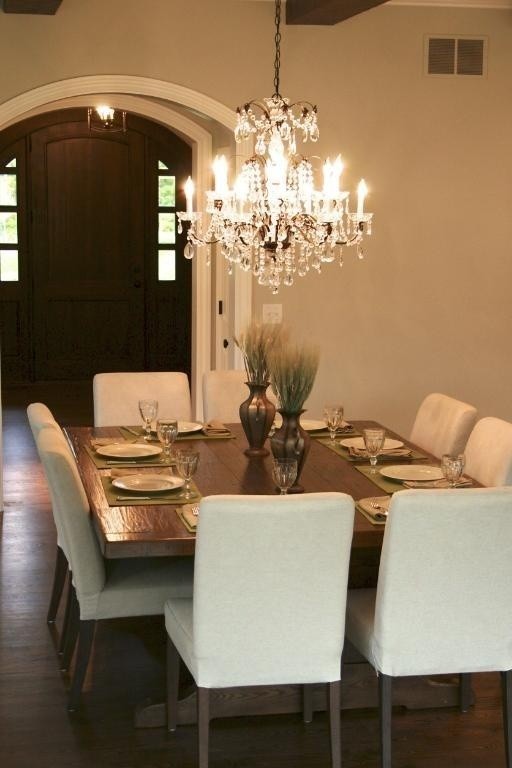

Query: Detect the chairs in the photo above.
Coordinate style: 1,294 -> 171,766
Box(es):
34,425 -> 189,703
27,402 -> 90,670
343,488 -> 512,768
202,371 -> 281,424
91,371 -> 192,429
161,491 -> 356,764
459,417 -> 511,491
409,392 -> 477,467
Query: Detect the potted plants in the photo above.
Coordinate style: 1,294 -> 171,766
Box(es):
235,321 -> 281,462
265,340 -> 317,493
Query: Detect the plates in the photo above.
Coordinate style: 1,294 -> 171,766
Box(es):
271,419 -> 328,430
340,437 -> 404,449
143,420 -> 203,432
113,474 -> 185,494
380,465 -> 445,480
96,443 -> 162,458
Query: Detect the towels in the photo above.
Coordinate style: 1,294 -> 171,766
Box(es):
181,502 -> 200,530
109,466 -> 173,481
401,476 -> 474,491
348,445 -> 414,459
335,420 -> 356,434
358,496 -> 392,522
89,437 -> 138,449
202,421 -> 232,436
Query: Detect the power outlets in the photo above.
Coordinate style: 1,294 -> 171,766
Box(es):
262,303 -> 285,327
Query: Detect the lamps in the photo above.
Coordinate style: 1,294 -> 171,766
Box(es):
87,104 -> 130,134
175,1 -> 373,294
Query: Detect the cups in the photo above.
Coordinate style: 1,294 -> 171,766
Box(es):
324,400 -> 344,444
272,459 -> 297,495
156,419 -> 178,461
175,449 -> 200,499
139,399 -> 158,440
363,428 -> 385,475
442,455 -> 466,488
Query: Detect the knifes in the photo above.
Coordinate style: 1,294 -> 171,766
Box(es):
116,495 -> 182,501
121,426 -> 140,435
106,460 -> 162,464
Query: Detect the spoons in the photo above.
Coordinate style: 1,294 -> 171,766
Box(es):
177,489 -> 197,499
369,501 -> 388,512
